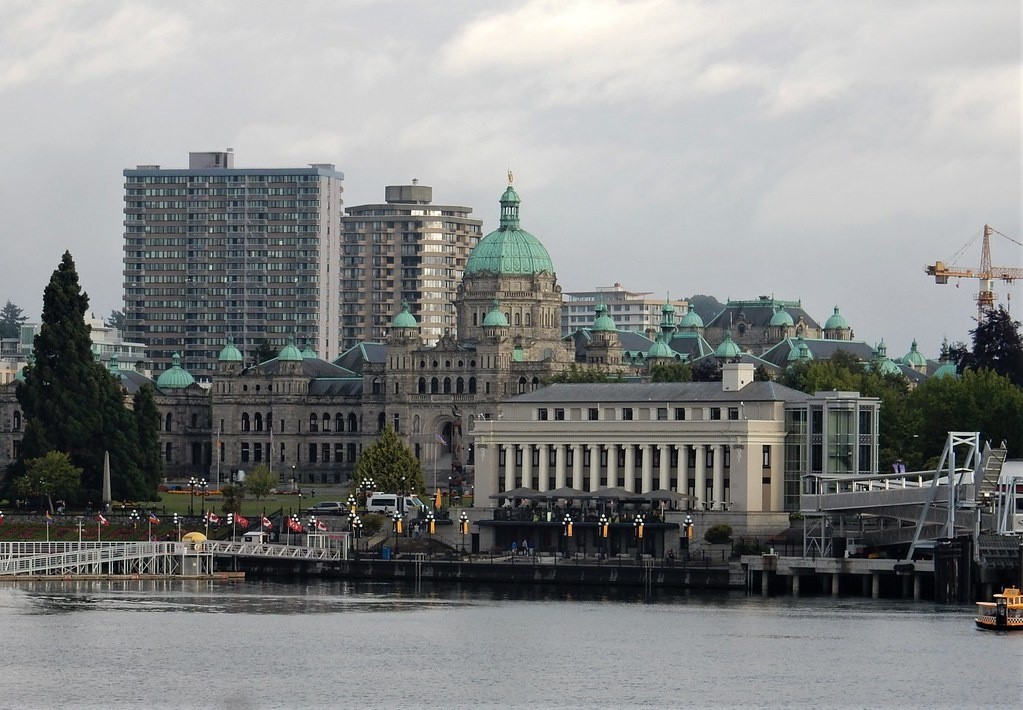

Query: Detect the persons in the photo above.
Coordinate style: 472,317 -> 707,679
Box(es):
443,487 -> 460,508
56,498 -> 157,514
493,504 -> 659,523
385,505 -> 390,517
14,500 -> 21,515
152,529 -> 186,554
298,487 -> 316,498
664,548 -> 676,568
408,521 -> 425,539
228,523 -> 234,542
268,531 -> 279,542
511,537 -> 534,555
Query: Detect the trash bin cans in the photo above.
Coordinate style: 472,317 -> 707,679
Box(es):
383,546 -> 390,559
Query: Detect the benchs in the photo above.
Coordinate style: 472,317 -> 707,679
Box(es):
113,504 -> 140,516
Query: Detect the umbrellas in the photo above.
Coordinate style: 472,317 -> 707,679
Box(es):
489,487 -> 698,518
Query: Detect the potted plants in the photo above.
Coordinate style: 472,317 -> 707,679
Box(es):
737,541 -> 771,563
702,524 -> 733,564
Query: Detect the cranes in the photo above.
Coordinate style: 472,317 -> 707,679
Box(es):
923,224 -> 1023,355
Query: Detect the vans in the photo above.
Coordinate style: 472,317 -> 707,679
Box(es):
366,491 -> 430,516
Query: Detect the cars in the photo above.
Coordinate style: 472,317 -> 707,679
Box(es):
158,486 -> 182,492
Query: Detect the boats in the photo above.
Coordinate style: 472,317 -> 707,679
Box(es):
974,585 -> 1023,631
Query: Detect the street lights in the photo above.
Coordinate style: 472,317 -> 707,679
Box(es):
308,515 -> 316,546
411,486 -> 414,496
683,515 -> 694,562
173,512 -> 178,540
292,465 -> 296,492
298,493 -> 302,518
598,514 -> 609,558
448,476 -> 452,507
203,512 -> 208,540
470,485 -> 474,507
187,477 -> 197,517
76,516 -> 85,542
346,494 -> 363,561
129,510 -> 140,535
227,513 -> 233,541
562,513 -> 574,558
392,511 -> 403,554
292,514 -> 299,546
633,515 -> 645,562
402,476 -> 405,497
458,511 -> 468,553
198,477 -> 209,516
425,511 -> 435,561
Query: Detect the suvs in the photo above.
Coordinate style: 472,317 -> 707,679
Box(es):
302,501 -> 348,516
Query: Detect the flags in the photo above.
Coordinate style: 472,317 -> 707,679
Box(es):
263,517 -> 272,529
97,511 -> 109,526
270,427 -> 276,454
46,511 -> 52,524
234,511 -> 249,528
316,519 -> 326,531
436,487 -> 442,520
287,517 -> 302,532
149,514 -> 160,524
208,511 -> 221,526
435,431 -> 447,446
